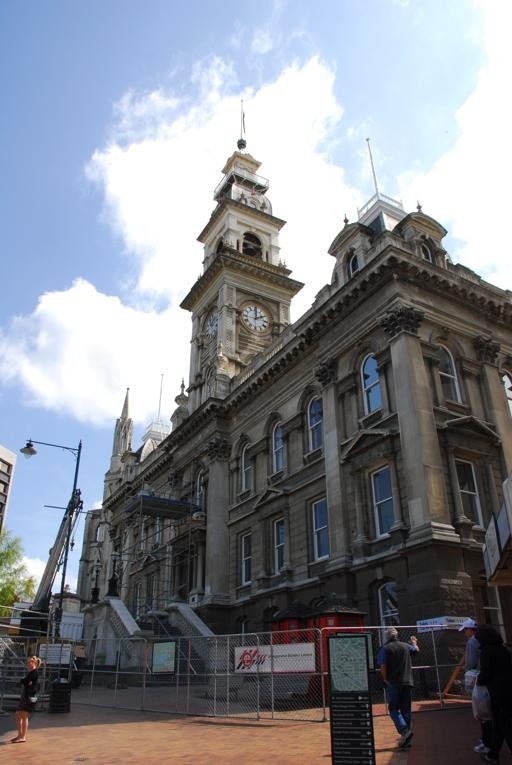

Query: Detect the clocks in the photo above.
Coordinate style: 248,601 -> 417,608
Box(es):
202,306 -> 219,342
238,299 -> 274,337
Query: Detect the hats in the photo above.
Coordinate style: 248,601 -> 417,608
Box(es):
458,618 -> 478,631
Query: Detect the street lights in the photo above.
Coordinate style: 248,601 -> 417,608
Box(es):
19,438 -> 82,641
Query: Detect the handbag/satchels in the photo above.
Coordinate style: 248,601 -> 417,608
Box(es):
462,670 -> 479,693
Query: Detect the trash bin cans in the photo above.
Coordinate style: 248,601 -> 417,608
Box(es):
47,682 -> 71,713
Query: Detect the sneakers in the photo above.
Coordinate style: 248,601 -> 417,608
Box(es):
399,728 -> 414,748
473,739 -> 500,765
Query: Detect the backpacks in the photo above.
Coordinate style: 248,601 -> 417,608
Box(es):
471,678 -> 492,722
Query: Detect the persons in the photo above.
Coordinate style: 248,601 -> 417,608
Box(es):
376,627 -> 422,755
458,618 -> 490,753
470,627 -> 512,765
10,656 -> 42,743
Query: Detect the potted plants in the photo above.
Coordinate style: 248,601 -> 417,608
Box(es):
0,577 -> 18,624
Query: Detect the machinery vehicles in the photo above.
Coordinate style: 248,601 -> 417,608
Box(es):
1,490 -> 82,689
